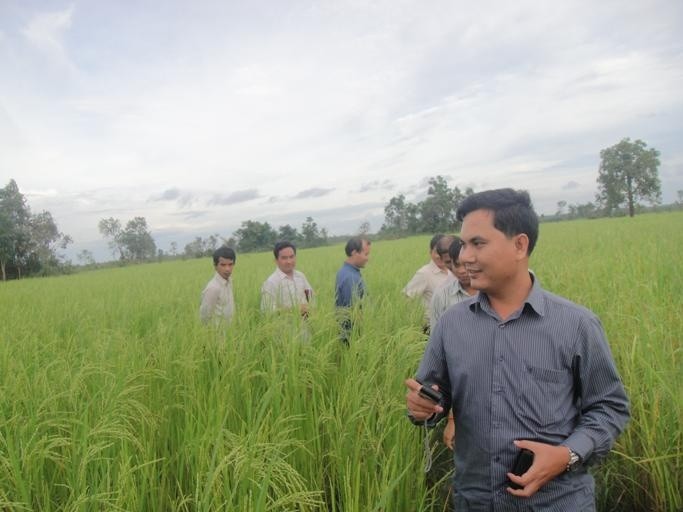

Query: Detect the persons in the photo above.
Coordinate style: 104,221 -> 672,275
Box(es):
401,235 -> 448,310
197,244 -> 237,320
398,184 -> 629,511
258,239 -> 315,347
430,235 -> 458,304
425,240 -> 479,336
333,237 -> 374,352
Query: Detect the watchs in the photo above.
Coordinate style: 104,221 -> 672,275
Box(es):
566,447 -> 579,476
